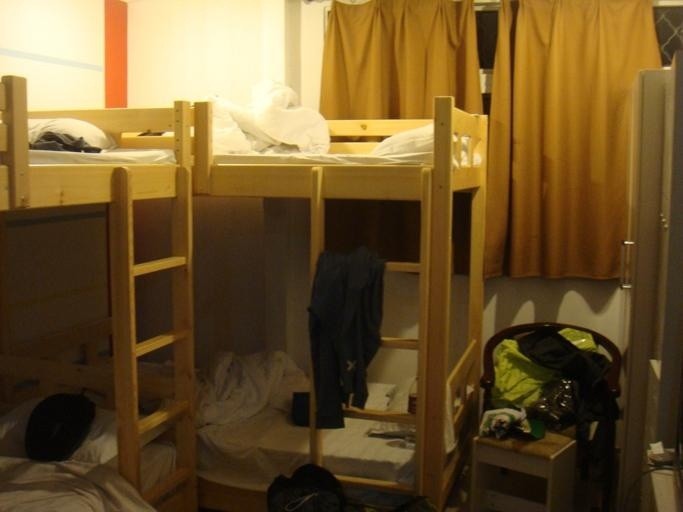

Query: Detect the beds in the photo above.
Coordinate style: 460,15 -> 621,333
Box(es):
189,96 -> 491,512
0,73 -> 199,512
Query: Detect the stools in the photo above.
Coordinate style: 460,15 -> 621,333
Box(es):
472,425 -> 578,512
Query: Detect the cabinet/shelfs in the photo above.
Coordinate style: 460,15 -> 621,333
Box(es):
617,67 -> 670,512
639,49 -> 683,512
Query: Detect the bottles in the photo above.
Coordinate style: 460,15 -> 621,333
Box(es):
405,371 -> 418,448
